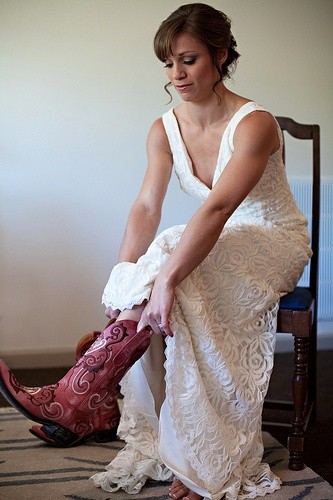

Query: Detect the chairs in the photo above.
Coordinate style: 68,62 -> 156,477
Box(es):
261,116 -> 322,472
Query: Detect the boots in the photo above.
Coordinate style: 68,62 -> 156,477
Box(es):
27,402 -> 123,445
0,319 -> 153,440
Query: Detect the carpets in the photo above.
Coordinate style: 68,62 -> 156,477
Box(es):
0,407 -> 332,500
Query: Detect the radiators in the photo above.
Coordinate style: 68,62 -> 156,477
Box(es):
286,180 -> 333,324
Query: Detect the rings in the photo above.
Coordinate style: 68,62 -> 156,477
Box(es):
157,323 -> 164,328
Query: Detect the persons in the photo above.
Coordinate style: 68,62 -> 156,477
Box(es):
0,3 -> 314,499
